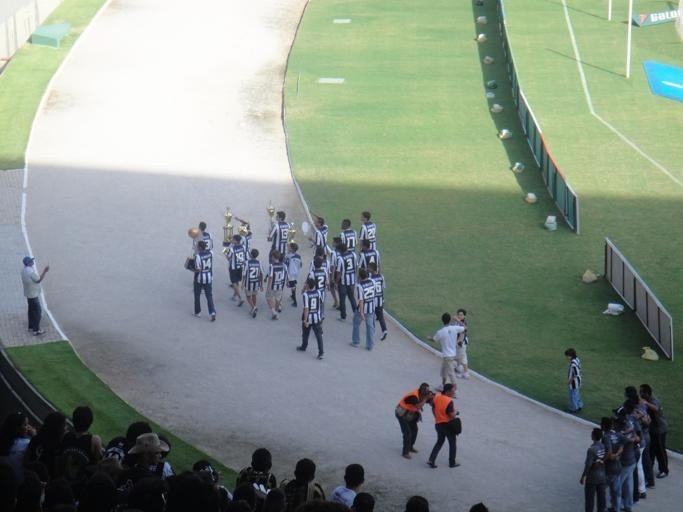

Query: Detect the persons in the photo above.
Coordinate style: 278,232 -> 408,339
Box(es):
190,211 -> 387,359
21,256 -> 49,336
427,383 -> 460,468
404,495 -> 429,512
426,313 -> 467,398
470,503 -> 488,512
564,348 -> 584,413
580,384 -> 669,512
450,309 -> 469,380
395,383 -> 432,459
1,406 -> 375,511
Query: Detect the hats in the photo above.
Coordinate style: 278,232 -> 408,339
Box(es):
126,433 -> 170,454
23,257 -> 34,263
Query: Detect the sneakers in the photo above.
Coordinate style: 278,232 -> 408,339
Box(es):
296,346 -> 305,351
192,312 -> 201,317
210,312 -> 215,320
606,472 -> 667,512
317,354 -> 324,359
454,367 -> 470,378
403,448 -> 461,467
332,303 -> 387,350
229,295 -> 297,319
29,327 -> 44,335
